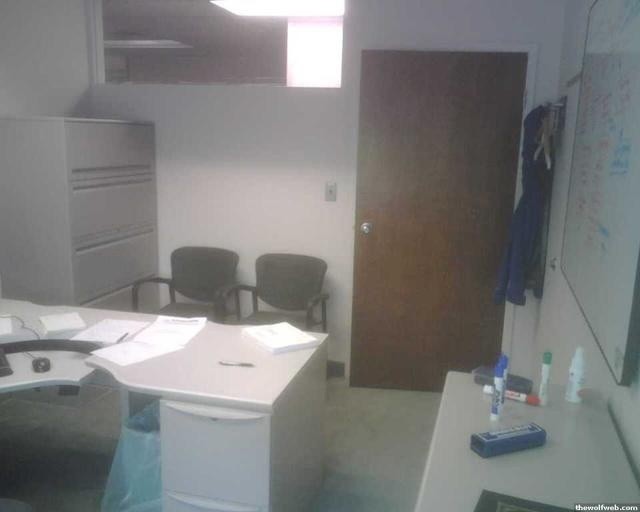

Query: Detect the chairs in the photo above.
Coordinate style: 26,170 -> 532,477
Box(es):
132,247 -> 240,324
220,253 -> 328,333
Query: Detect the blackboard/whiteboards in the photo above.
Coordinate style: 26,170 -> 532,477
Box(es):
559,2 -> 640,387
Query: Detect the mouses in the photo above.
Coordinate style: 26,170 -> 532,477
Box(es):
32,357 -> 50,373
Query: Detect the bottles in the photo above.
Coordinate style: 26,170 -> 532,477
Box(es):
483,347 -> 586,421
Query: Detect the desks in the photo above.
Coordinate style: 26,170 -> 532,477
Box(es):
415,370 -> 640,512
0,298 -> 329,512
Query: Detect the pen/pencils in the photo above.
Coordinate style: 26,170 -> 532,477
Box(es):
482,351 -> 552,420
117,332 -> 128,344
219,361 -> 252,367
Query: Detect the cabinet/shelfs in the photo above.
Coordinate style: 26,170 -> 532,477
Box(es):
0,115 -> 160,408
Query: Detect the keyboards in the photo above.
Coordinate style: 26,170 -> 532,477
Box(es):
0,349 -> 13,377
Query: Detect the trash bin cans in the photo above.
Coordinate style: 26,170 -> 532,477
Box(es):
99,399 -> 162,511
